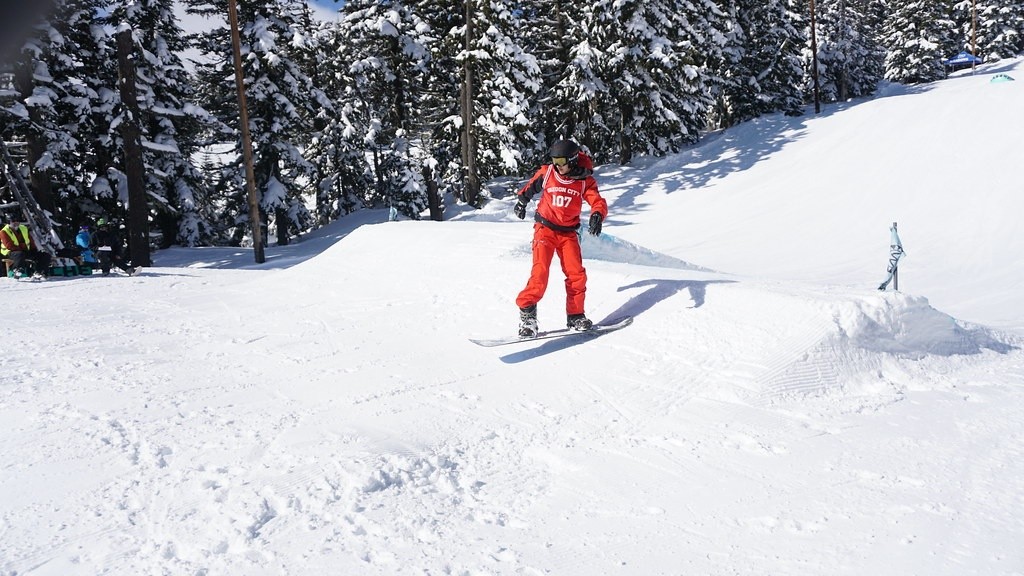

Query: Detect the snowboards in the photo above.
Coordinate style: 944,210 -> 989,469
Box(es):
466,315 -> 634,348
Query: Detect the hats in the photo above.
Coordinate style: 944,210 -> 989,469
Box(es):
97,218 -> 109,227
79,222 -> 89,229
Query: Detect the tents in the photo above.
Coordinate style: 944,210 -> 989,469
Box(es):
943,51 -> 982,80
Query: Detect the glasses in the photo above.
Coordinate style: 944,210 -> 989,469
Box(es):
12,220 -> 19,222
552,154 -> 578,167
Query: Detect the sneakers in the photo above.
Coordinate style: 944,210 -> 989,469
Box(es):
13,267 -> 22,279
518,303 -> 538,339
31,272 -> 46,281
567,313 -> 592,331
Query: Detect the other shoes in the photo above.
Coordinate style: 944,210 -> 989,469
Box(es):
125,266 -> 135,274
103,268 -> 110,276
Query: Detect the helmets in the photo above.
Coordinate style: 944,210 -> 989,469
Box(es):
550,140 -> 579,168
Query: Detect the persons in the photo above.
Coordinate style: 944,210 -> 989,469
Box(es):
75,217 -> 141,277
0,213 -> 51,282
514,140 -> 608,340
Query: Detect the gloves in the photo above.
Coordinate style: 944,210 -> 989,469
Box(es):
588,211 -> 602,235
514,200 -> 527,219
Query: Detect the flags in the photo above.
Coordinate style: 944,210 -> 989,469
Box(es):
877,228 -> 906,289
